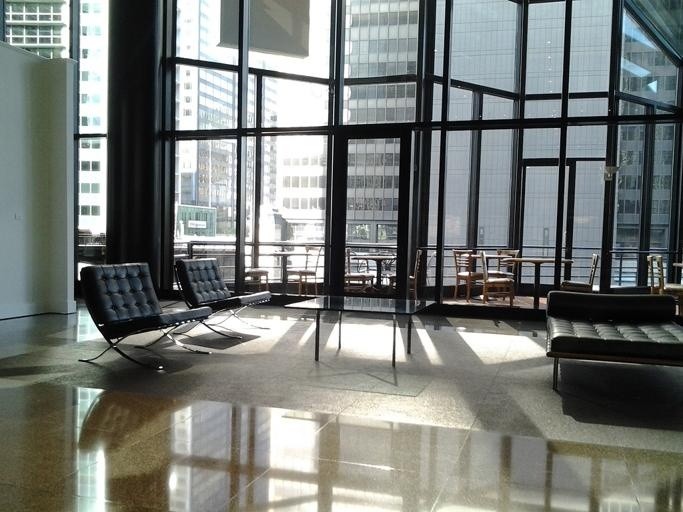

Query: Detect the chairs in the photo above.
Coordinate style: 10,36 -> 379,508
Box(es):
453,249 -> 519,306
244,244 -> 423,299
647,256 -> 660,294
561,254 -> 598,292
656,256 -> 683,316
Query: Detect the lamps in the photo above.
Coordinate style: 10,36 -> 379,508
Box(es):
600,166 -> 620,181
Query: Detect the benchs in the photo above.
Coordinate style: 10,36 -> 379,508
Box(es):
544,289 -> 683,390
79,262 -> 212,371
174,258 -> 273,339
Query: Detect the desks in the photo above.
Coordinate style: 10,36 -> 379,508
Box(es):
673,263 -> 683,267
504,258 -> 573,307
